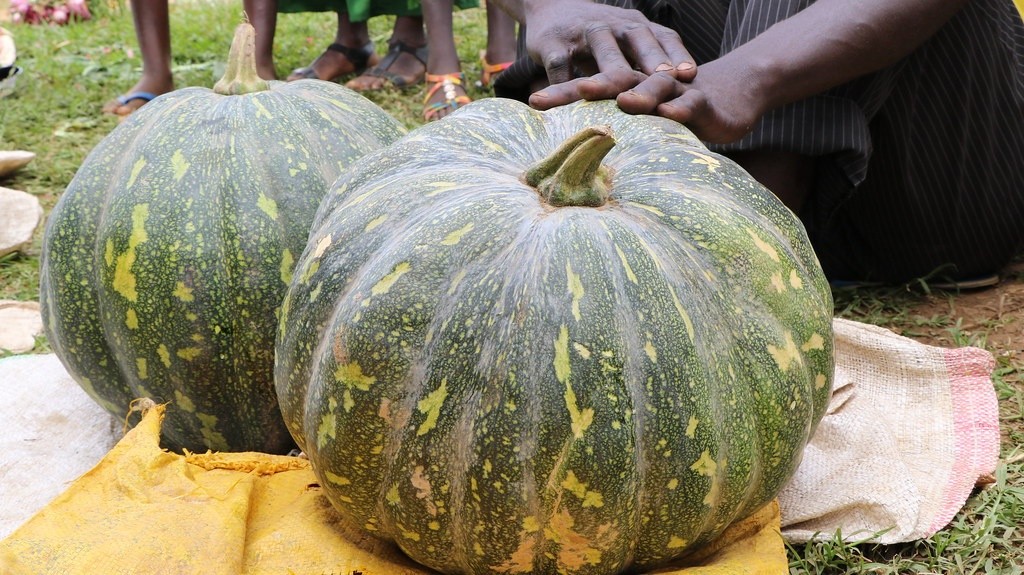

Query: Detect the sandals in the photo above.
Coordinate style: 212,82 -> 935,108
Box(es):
348,42 -> 429,92
287,40 -> 376,82
479,47 -> 517,93
424,72 -> 472,122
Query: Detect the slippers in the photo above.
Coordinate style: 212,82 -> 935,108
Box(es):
115,92 -> 157,121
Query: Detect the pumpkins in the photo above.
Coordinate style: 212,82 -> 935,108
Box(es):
36,24 -> 412,453
274,98 -> 835,575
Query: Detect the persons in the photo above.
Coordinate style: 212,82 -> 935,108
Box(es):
492,0 -> 1023,290
102,0 -> 518,126
